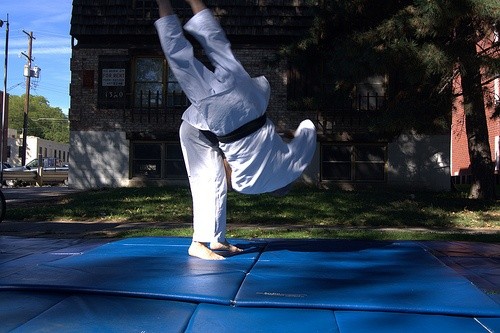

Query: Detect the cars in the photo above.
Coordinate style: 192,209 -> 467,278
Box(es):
0,157 -> 69,185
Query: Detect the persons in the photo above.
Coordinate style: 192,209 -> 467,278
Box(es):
154,1 -> 316,197
178,104 -> 243,259
